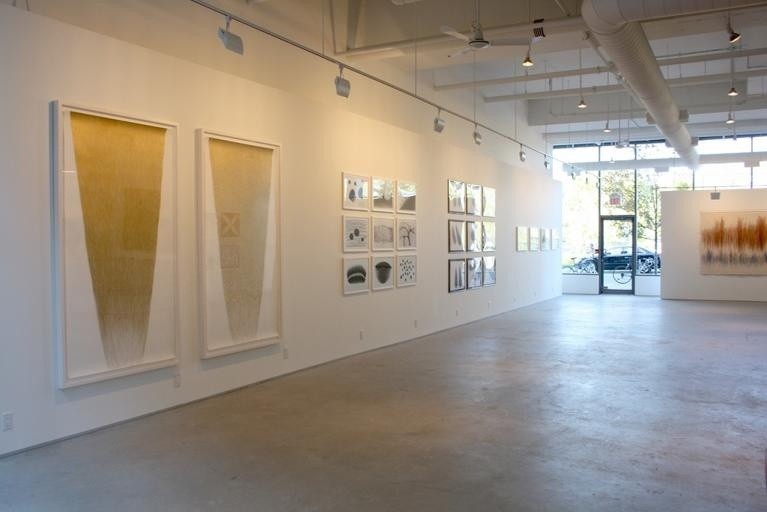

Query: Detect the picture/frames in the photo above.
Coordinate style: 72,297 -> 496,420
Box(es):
341,172 -> 416,295
448,179 -> 496,292
52,97 -> 180,390
196,128 -> 283,360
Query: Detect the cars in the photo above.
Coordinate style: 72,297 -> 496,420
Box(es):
574,245 -> 662,271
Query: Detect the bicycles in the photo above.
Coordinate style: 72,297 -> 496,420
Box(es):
612,255 -> 661,285
562,256 -> 599,274
